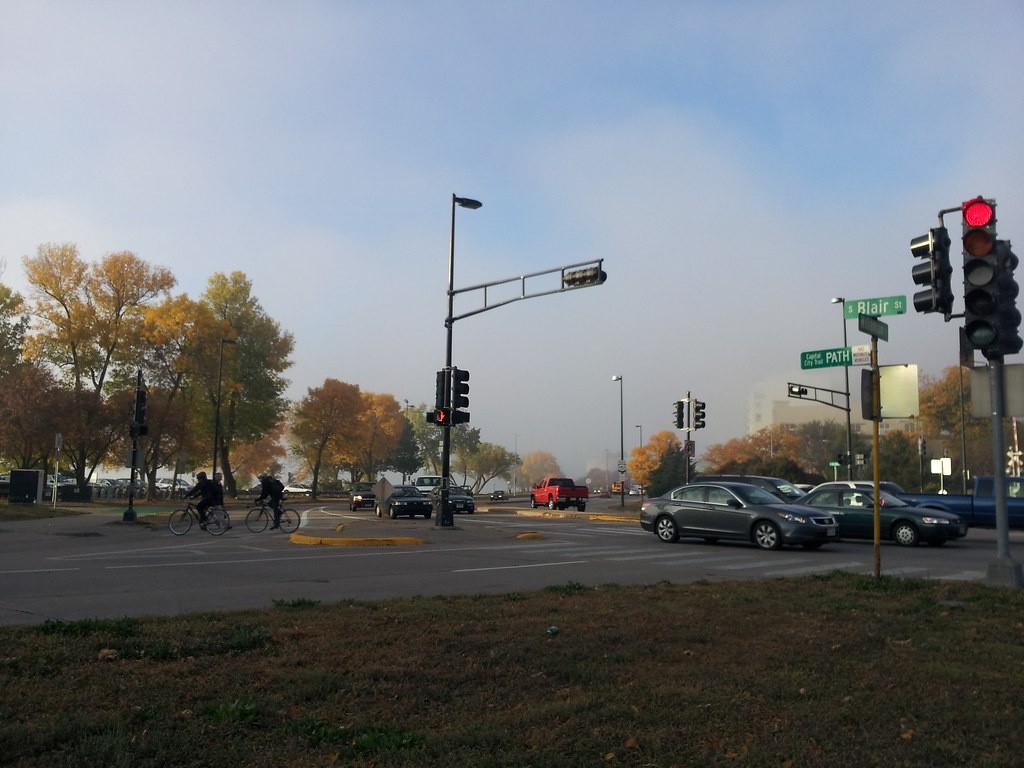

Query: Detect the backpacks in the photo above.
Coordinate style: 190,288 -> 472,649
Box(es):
268,476 -> 284,492
203,478 -> 222,496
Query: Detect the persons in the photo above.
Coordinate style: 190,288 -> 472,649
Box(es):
185,472 -> 218,530
213,473 -> 232,530
255,472 -> 283,531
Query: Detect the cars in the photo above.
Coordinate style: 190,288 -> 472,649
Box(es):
245,484 -> 263,494
348,483 -> 377,512
0,473 -> 195,494
490,491 -> 509,501
374,486 -> 433,519
625,485 -> 645,496
428,484 -> 475,514
639,475 -> 967,550
282,483 -> 312,496
599,491 -> 610,498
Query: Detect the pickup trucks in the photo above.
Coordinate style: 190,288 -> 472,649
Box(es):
530,477 -> 589,512
896,474 -> 1023,529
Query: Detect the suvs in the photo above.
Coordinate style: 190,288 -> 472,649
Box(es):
414,476 -> 443,496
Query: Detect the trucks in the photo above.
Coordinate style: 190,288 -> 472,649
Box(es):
611,481 -> 626,495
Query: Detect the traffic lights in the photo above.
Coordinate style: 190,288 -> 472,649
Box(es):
435,410 -> 449,424
564,269 -> 606,285
454,411 -> 470,425
910,227 -> 954,314
790,386 -> 809,395
453,369 -> 469,408
838,453 -> 866,466
693,399 -> 705,429
962,198 -> 1023,355
673,401 -> 683,429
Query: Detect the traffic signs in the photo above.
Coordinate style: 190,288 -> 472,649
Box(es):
843,294 -> 907,342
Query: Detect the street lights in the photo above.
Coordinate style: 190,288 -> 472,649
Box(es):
832,298 -> 853,480
613,375 -> 625,507
213,339 -> 235,481
435,194 -> 482,525
636,425 -> 642,448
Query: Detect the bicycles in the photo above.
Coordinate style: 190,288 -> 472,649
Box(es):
245,498 -> 301,534
168,496 -> 231,536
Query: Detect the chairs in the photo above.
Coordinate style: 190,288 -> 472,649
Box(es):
710,494 -> 718,501
357,486 -> 362,490
844,498 -> 851,505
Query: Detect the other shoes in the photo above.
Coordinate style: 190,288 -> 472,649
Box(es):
200,521 -> 208,526
271,526 -> 278,530
227,526 -> 233,529
